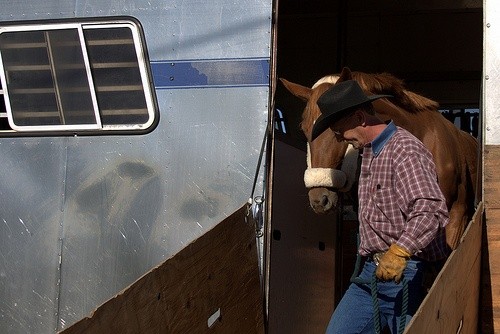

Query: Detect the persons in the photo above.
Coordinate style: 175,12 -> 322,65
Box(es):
310,80 -> 449,334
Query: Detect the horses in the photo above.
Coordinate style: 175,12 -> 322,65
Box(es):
278,67 -> 484,262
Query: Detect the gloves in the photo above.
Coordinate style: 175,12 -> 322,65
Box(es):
374,242 -> 413,285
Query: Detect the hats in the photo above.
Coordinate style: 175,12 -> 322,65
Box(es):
310,79 -> 393,143
